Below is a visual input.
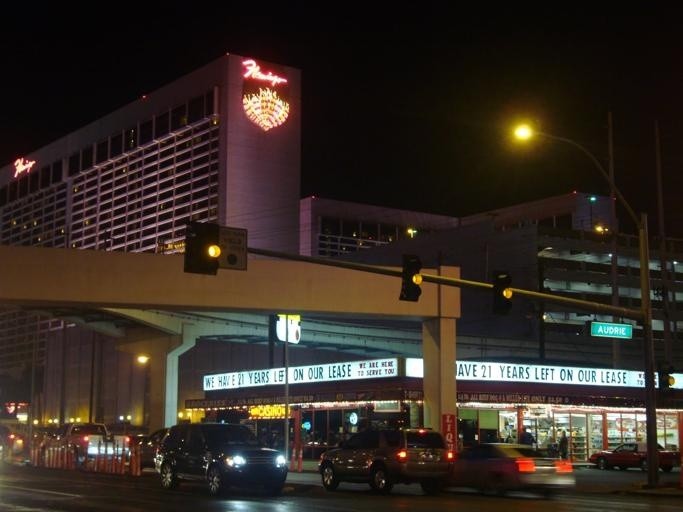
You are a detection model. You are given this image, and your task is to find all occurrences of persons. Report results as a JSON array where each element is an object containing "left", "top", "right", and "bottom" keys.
[
  {"left": 501, "top": 420, "right": 512, "bottom": 443},
  {"left": 520, "top": 426, "right": 537, "bottom": 445},
  {"left": 547, "top": 423, "right": 557, "bottom": 441},
  {"left": 558, "top": 431, "right": 569, "bottom": 459}
]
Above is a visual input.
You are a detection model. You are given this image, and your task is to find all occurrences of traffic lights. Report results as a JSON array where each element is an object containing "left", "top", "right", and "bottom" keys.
[
  {"left": 492, "top": 270, "right": 513, "bottom": 316},
  {"left": 399, "top": 254, "right": 423, "bottom": 302},
  {"left": 183, "top": 221, "right": 221, "bottom": 276},
  {"left": 658, "top": 361, "right": 675, "bottom": 393}
]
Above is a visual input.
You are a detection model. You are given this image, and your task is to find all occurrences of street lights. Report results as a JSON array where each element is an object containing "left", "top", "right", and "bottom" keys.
[
  {"left": 513, "top": 120, "right": 658, "bottom": 488},
  {"left": 594, "top": 220, "right": 638, "bottom": 240}
]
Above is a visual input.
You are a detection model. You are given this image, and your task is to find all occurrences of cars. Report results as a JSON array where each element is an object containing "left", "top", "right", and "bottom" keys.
[
  {"left": 0, "top": 419, "right": 287, "bottom": 496},
  {"left": 590, "top": 442, "right": 680, "bottom": 472},
  {"left": 318, "top": 426, "right": 576, "bottom": 496}
]
